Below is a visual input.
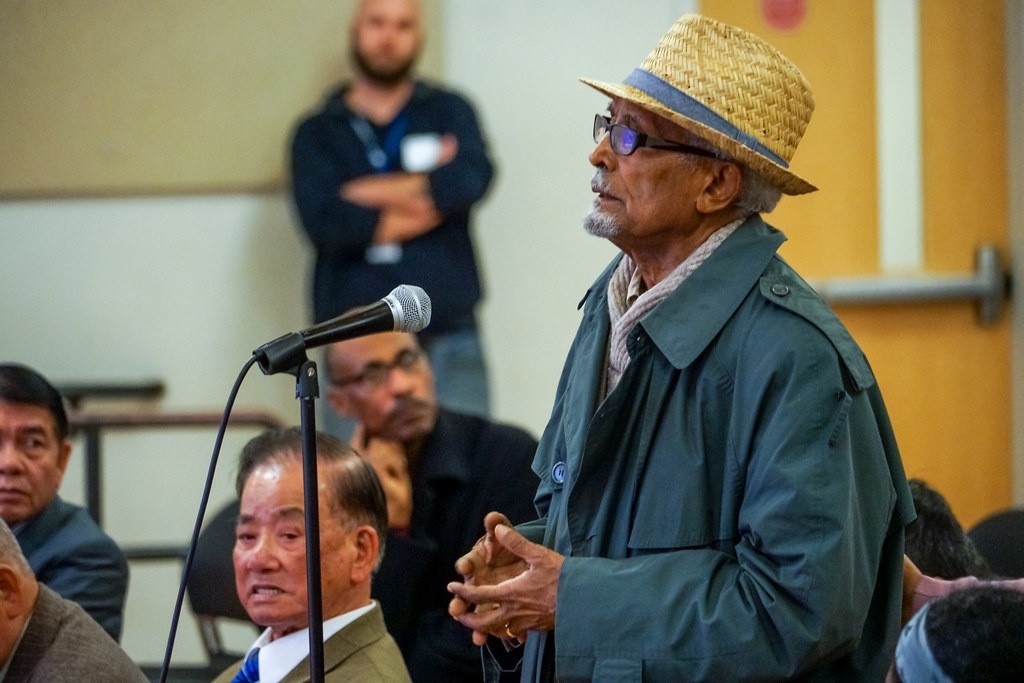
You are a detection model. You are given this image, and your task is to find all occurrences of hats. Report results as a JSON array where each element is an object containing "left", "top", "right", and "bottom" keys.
[{"left": 578, "top": 13, "right": 821, "bottom": 196}]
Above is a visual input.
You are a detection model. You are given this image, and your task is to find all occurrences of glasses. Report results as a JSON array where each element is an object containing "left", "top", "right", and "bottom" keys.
[
  {"left": 593, "top": 114, "right": 737, "bottom": 163},
  {"left": 337, "top": 352, "right": 420, "bottom": 384}
]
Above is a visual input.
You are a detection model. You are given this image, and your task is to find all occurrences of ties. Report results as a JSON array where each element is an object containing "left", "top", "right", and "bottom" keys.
[{"left": 230, "top": 647, "right": 259, "bottom": 683}]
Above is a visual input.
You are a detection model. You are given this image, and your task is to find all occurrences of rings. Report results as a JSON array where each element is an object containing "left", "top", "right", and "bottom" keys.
[{"left": 504, "top": 621, "right": 516, "bottom": 638}]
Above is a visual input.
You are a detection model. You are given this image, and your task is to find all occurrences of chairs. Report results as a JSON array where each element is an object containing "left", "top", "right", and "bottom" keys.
[{"left": 187, "top": 500, "right": 264, "bottom": 683}]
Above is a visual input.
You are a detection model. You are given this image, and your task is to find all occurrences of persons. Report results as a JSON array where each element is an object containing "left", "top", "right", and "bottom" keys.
[
  {"left": 209, "top": 425, "right": 411, "bottom": 683},
  {"left": 325, "top": 306, "right": 544, "bottom": 683},
  {"left": 286, "top": 0, "right": 497, "bottom": 446},
  {"left": 906, "top": 474, "right": 1024, "bottom": 683},
  {"left": 0, "top": 361, "right": 149, "bottom": 683},
  {"left": 446, "top": 13, "right": 918, "bottom": 683}
]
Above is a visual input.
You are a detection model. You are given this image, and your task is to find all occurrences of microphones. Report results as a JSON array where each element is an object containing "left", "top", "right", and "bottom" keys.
[{"left": 251, "top": 283, "right": 432, "bottom": 362}]
